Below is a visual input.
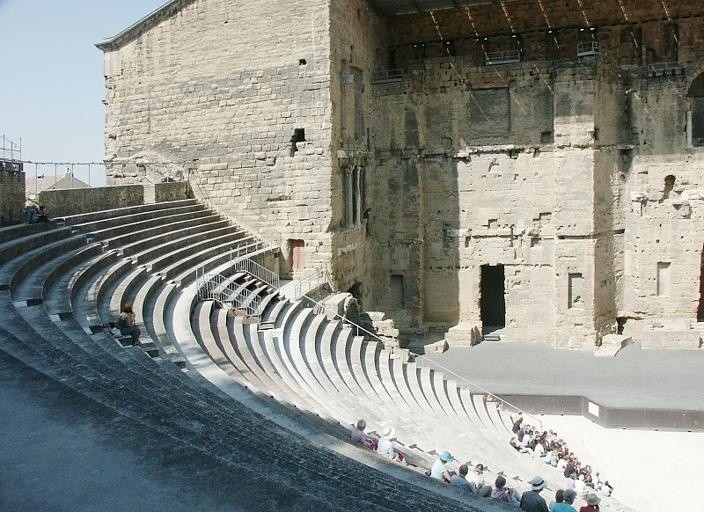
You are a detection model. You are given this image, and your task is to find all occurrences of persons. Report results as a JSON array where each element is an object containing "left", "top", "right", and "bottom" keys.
[
  {"left": 432, "top": 451, "right": 452, "bottom": 485},
  {"left": 113, "top": 304, "right": 141, "bottom": 346},
  {"left": 449, "top": 465, "right": 474, "bottom": 492},
  {"left": 491, "top": 477, "right": 521, "bottom": 508},
  {"left": 348, "top": 419, "right": 377, "bottom": 449},
  {"left": 510, "top": 416, "right": 613, "bottom": 512},
  {"left": 475, "top": 463, "right": 492, "bottom": 497},
  {"left": 376, "top": 426, "right": 405, "bottom": 463},
  {"left": 37, "top": 205, "right": 48, "bottom": 222}
]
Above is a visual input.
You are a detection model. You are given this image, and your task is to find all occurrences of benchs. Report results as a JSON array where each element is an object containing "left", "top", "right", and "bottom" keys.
[{"left": 0, "top": 197, "right": 621, "bottom": 512}]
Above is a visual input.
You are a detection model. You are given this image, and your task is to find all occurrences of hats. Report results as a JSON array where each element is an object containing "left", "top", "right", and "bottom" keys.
[
  {"left": 440, "top": 452, "right": 453, "bottom": 462},
  {"left": 383, "top": 427, "right": 394, "bottom": 439},
  {"left": 587, "top": 494, "right": 601, "bottom": 505},
  {"left": 532, "top": 476, "right": 544, "bottom": 491}
]
